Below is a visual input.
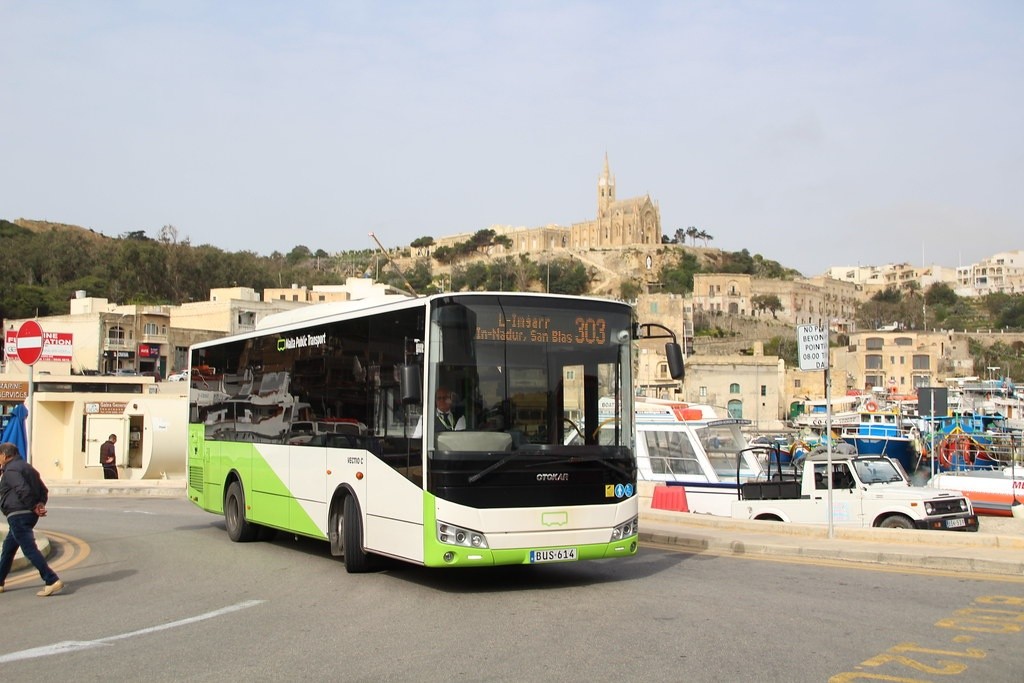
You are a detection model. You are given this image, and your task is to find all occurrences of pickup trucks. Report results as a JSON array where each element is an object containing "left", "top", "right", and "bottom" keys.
[{"left": 732, "top": 444, "right": 980, "bottom": 535}]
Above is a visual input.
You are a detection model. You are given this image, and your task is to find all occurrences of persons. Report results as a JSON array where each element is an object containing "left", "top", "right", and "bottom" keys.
[
  {"left": 100, "top": 434, "right": 118, "bottom": 480},
  {"left": 412, "top": 388, "right": 467, "bottom": 453},
  {"left": 0, "top": 443, "right": 64, "bottom": 596}
]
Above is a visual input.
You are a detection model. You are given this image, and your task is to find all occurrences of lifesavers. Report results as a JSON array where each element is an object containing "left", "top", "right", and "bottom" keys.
[
  {"left": 818, "top": 420, "right": 822, "bottom": 425},
  {"left": 958, "top": 437, "right": 971, "bottom": 462},
  {"left": 939, "top": 441, "right": 951, "bottom": 468},
  {"left": 866, "top": 401, "right": 878, "bottom": 413}
]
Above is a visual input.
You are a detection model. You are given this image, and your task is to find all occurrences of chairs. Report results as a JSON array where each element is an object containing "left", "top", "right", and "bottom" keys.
[
  {"left": 832, "top": 472, "right": 844, "bottom": 489},
  {"left": 816, "top": 472, "right": 827, "bottom": 489}
]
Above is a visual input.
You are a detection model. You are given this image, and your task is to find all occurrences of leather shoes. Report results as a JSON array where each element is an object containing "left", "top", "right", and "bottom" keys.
[
  {"left": 0, "top": 586, "right": 4, "bottom": 593},
  {"left": 37, "top": 580, "right": 64, "bottom": 596}
]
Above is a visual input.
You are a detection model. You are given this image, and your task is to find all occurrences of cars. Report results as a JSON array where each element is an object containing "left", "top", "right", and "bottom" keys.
[
  {"left": 111, "top": 368, "right": 138, "bottom": 376},
  {"left": 169, "top": 369, "right": 188, "bottom": 381},
  {"left": 137, "top": 371, "right": 161, "bottom": 383},
  {"left": 81, "top": 370, "right": 100, "bottom": 376}
]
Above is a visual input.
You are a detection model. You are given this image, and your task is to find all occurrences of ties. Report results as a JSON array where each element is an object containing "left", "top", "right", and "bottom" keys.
[{"left": 442, "top": 412, "right": 451, "bottom": 428}]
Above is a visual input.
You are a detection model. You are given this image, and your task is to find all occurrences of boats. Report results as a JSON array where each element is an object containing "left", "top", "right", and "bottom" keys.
[{"left": 563, "top": 376, "right": 1024, "bottom": 520}]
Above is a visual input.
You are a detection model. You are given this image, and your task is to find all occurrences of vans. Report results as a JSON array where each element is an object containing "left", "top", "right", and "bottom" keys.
[{"left": 289, "top": 417, "right": 367, "bottom": 447}]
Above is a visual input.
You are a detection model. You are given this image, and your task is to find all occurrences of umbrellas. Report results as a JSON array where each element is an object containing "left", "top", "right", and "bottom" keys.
[{"left": 0, "top": 404, "right": 28, "bottom": 463}]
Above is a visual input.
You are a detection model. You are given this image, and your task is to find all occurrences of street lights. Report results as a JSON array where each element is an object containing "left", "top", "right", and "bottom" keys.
[{"left": 115, "top": 312, "right": 130, "bottom": 372}]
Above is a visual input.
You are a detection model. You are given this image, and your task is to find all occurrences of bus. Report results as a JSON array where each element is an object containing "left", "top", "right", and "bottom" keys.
[{"left": 185, "top": 292, "right": 689, "bottom": 575}]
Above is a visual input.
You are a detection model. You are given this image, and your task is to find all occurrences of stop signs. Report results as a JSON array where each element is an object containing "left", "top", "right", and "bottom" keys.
[{"left": 15, "top": 321, "right": 44, "bottom": 366}]
